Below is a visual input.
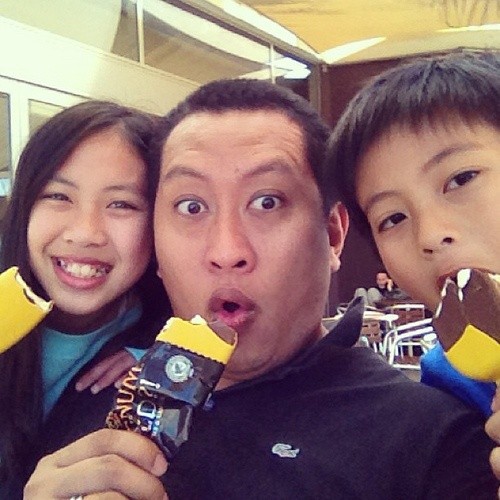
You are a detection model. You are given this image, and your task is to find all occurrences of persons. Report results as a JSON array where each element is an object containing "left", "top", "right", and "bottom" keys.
[
  {"left": 0, "top": 100, "right": 175, "bottom": 500},
  {"left": 326, "top": 45, "right": 499, "bottom": 499},
  {"left": 22, "top": 79, "right": 500, "bottom": 500},
  {"left": 353, "top": 270, "right": 398, "bottom": 312}
]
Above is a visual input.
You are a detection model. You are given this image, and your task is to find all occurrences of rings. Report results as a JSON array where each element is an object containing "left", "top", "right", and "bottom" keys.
[{"left": 68, "top": 493, "right": 87, "bottom": 500}]
